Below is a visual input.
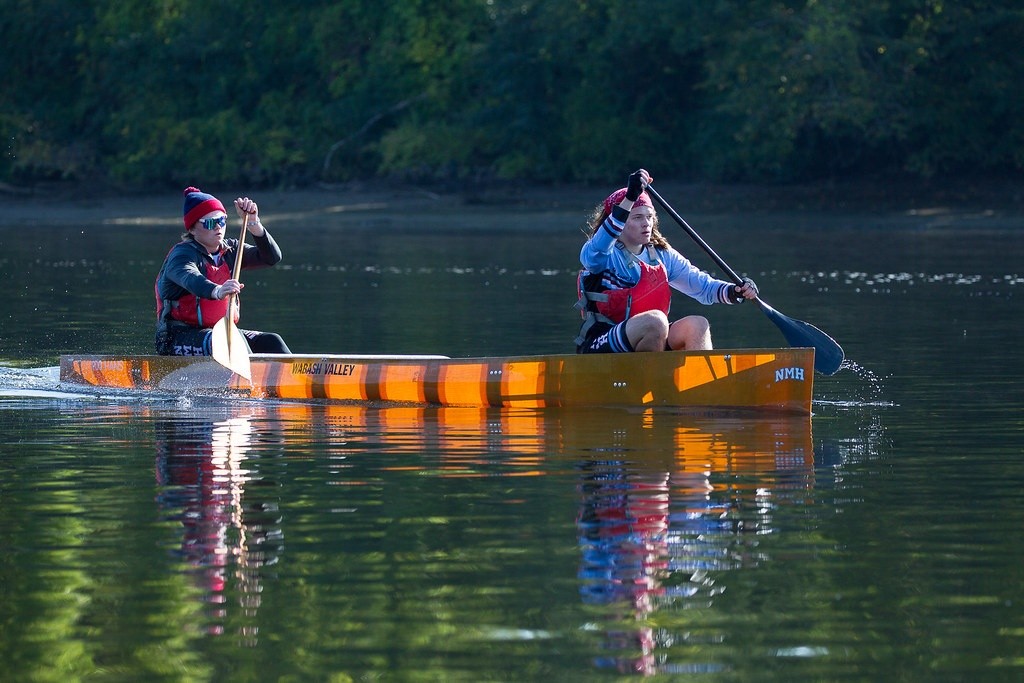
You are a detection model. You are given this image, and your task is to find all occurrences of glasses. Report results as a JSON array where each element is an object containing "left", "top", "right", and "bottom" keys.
[{"left": 199, "top": 215, "right": 227, "bottom": 230}]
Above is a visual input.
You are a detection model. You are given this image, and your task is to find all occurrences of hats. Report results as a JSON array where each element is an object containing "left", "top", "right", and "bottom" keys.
[{"left": 183, "top": 187, "right": 226, "bottom": 230}]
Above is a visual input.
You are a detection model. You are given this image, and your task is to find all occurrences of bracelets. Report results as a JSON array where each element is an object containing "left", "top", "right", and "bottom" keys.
[{"left": 247, "top": 220, "right": 259, "bottom": 226}]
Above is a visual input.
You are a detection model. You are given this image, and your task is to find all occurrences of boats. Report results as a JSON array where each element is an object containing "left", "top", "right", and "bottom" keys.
[{"left": 60, "top": 347, "right": 817, "bottom": 419}]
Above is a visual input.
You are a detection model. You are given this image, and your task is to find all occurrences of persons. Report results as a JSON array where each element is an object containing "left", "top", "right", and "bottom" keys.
[
  {"left": 155, "top": 187, "right": 292, "bottom": 354},
  {"left": 576, "top": 169, "right": 759, "bottom": 353}
]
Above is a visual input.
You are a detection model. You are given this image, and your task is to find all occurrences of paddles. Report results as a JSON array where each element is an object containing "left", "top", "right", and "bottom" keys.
[
  {"left": 209, "top": 205, "right": 256, "bottom": 383},
  {"left": 645, "top": 182, "right": 846, "bottom": 378}
]
